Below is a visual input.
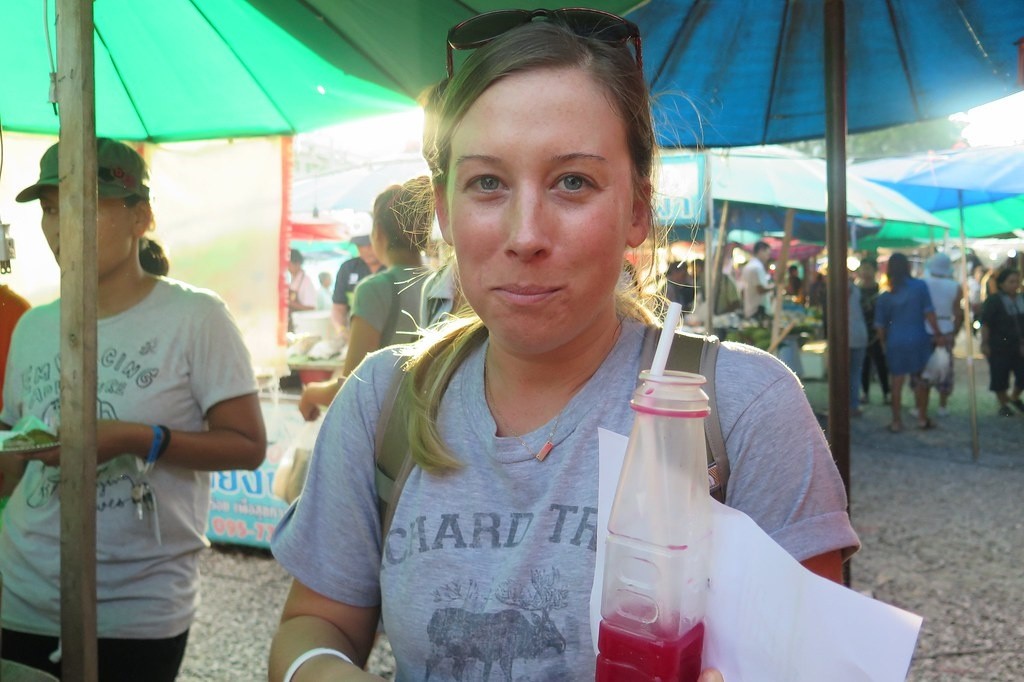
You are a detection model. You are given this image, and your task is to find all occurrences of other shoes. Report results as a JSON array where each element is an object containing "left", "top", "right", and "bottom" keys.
[
  {"left": 891, "top": 425, "right": 900, "bottom": 432},
  {"left": 884, "top": 392, "right": 892, "bottom": 403},
  {"left": 1009, "top": 397, "right": 1024, "bottom": 411},
  {"left": 859, "top": 393, "right": 868, "bottom": 403},
  {"left": 937, "top": 406, "right": 950, "bottom": 416},
  {"left": 1000, "top": 407, "right": 1012, "bottom": 416},
  {"left": 919, "top": 420, "right": 928, "bottom": 427},
  {"left": 909, "top": 408, "right": 920, "bottom": 418}
]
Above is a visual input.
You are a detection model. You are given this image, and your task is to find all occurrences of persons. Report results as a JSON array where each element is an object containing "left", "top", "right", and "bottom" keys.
[
  {"left": 285, "top": 175, "right": 1024, "bottom": 435},
  {"left": 0, "top": 136, "right": 267, "bottom": 682},
  {"left": 265, "top": 22, "right": 861, "bottom": 682}
]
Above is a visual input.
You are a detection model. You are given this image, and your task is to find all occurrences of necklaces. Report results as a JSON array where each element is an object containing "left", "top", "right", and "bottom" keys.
[{"left": 486, "top": 353, "right": 564, "bottom": 462}]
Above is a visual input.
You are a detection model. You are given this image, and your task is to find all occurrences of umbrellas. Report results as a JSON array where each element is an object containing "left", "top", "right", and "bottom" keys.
[
  {"left": 620, "top": 0, "right": 1024, "bottom": 251},
  {"left": 0, "top": 0, "right": 648, "bottom": 143}
]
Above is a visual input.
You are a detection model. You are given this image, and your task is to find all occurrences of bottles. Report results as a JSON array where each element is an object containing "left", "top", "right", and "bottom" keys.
[{"left": 595, "top": 370, "right": 711, "bottom": 682}]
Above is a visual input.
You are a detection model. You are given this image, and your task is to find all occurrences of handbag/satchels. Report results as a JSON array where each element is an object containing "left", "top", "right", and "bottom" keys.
[
  {"left": 847, "top": 286, "right": 869, "bottom": 348},
  {"left": 921, "top": 345, "right": 950, "bottom": 384},
  {"left": 272, "top": 443, "right": 314, "bottom": 505},
  {"left": 699, "top": 271, "right": 739, "bottom": 315}
]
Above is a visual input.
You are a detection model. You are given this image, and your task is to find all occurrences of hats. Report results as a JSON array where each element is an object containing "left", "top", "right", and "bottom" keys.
[
  {"left": 348, "top": 210, "right": 374, "bottom": 245},
  {"left": 928, "top": 253, "right": 954, "bottom": 274},
  {"left": 15, "top": 138, "right": 149, "bottom": 199}
]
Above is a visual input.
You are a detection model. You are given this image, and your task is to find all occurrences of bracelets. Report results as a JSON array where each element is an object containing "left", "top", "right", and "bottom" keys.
[
  {"left": 157, "top": 424, "right": 171, "bottom": 456},
  {"left": 136, "top": 423, "right": 162, "bottom": 475},
  {"left": 277, "top": 648, "right": 355, "bottom": 682}
]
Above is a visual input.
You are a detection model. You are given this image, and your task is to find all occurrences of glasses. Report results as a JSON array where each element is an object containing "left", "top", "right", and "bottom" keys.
[{"left": 447, "top": 7, "right": 644, "bottom": 79}]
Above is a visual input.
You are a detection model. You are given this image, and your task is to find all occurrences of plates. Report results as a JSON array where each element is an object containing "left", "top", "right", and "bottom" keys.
[{"left": 0, "top": 430, "right": 60, "bottom": 453}]
